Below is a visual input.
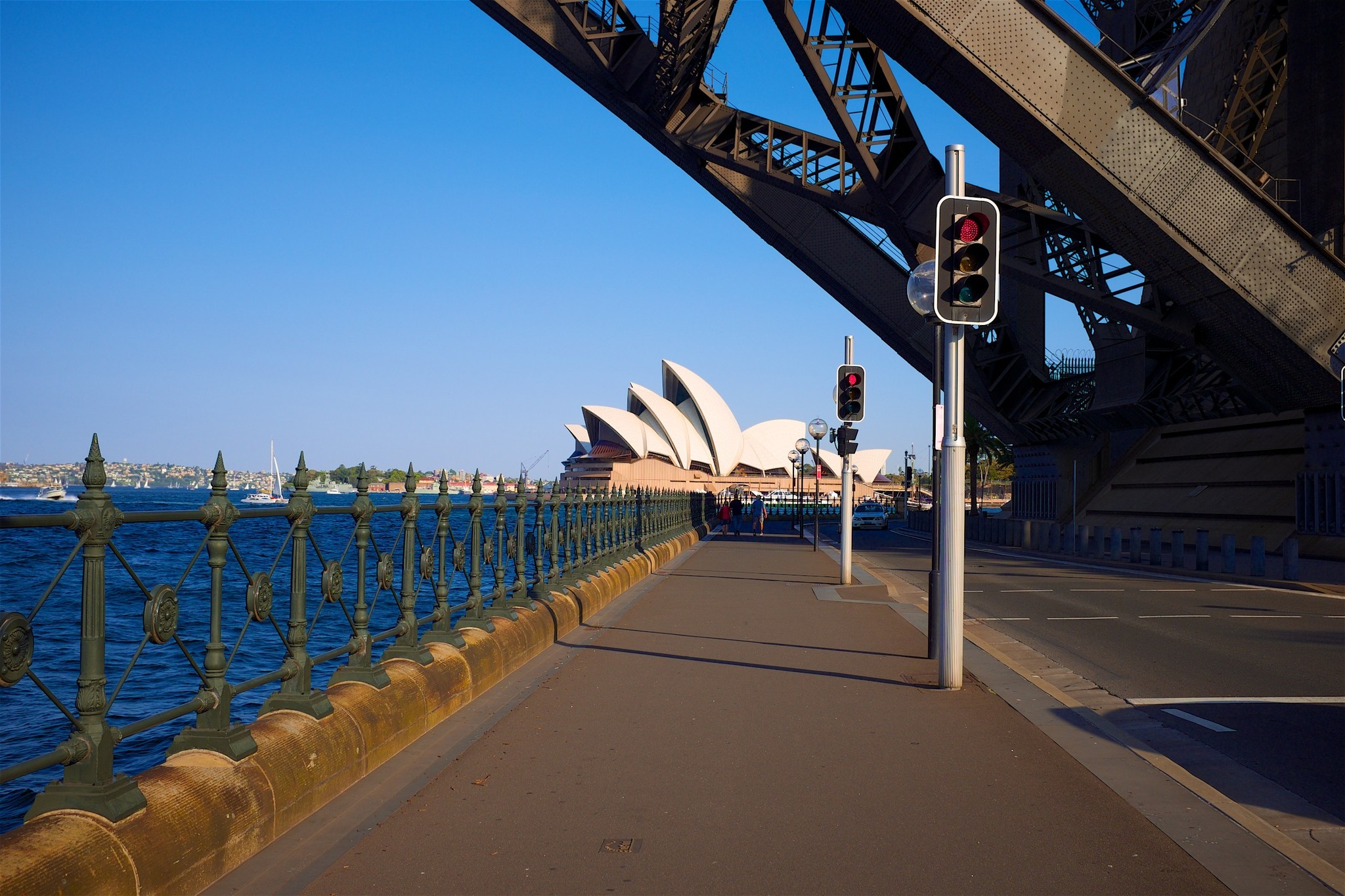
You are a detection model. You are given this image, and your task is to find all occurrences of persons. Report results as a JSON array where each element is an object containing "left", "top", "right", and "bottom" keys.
[
  {"left": 729, "top": 494, "right": 744, "bottom": 536},
  {"left": 751, "top": 495, "right": 768, "bottom": 537},
  {"left": 717, "top": 501, "right": 733, "bottom": 537}
]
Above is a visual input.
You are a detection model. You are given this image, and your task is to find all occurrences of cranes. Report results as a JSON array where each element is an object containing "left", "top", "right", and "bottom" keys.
[{"left": 520, "top": 450, "right": 549, "bottom": 484}]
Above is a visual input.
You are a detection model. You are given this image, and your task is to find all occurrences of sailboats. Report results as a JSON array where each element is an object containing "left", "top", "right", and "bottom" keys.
[{"left": 241, "top": 440, "right": 290, "bottom": 503}]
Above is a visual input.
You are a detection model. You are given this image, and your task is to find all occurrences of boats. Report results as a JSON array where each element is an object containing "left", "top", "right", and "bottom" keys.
[
  {"left": 327, "top": 489, "right": 340, "bottom": 494},
  {"left": 36, "top": 485, "right": 67, "bottom": 500}
]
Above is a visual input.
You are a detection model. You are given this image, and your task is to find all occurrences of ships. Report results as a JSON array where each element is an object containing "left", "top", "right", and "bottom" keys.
[{"left": 288, "top": 471, "right": 358, "bottom": 492}]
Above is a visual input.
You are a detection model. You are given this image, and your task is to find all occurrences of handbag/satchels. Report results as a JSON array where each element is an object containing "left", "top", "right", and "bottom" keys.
[{"left": 717, "top": 507, "right": 724, "bottom": 519}]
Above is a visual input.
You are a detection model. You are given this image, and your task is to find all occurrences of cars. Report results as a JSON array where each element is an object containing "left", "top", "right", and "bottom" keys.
[{"left": 852, "top": 503, "right": 889, "bottom": 530}]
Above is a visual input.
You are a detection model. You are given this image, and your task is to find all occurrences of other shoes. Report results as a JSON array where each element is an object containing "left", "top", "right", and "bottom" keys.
[
  {"left": 738, "top": 533, "right": 740, "bottom": 536},
  {"left": 760, "top": 532, "right": 763, "bottom": 537},
  {"left": 723, "top": 532, "right": 724, "bottom": 536},
  {"left": 735, "top": 535, "right": 737, "bottom": 537},
  {"left": 753, "top": 533, "right": 757, "bottom": 536},
  {"left": 725, "top": 532, "right": 727, "bottom": 536}
]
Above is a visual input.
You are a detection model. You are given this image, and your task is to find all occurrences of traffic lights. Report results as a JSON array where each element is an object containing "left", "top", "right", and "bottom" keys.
[
  {"left": 933, "top": 193, "right": 1000, "bottom": 326},
  {"left": 907, "top": 466, "right": 912, "bottom": 491},
  {"left": 833, "top": 364, "right": 865, "bottom": 457}
]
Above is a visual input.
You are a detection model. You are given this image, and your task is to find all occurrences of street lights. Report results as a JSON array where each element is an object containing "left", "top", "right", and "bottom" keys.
[
  {"left": 908, "top": 260, "right": 944, "bottom": 659},
  {"left": 788, "top": 417, "right": 827, "bottom": 551}
]
